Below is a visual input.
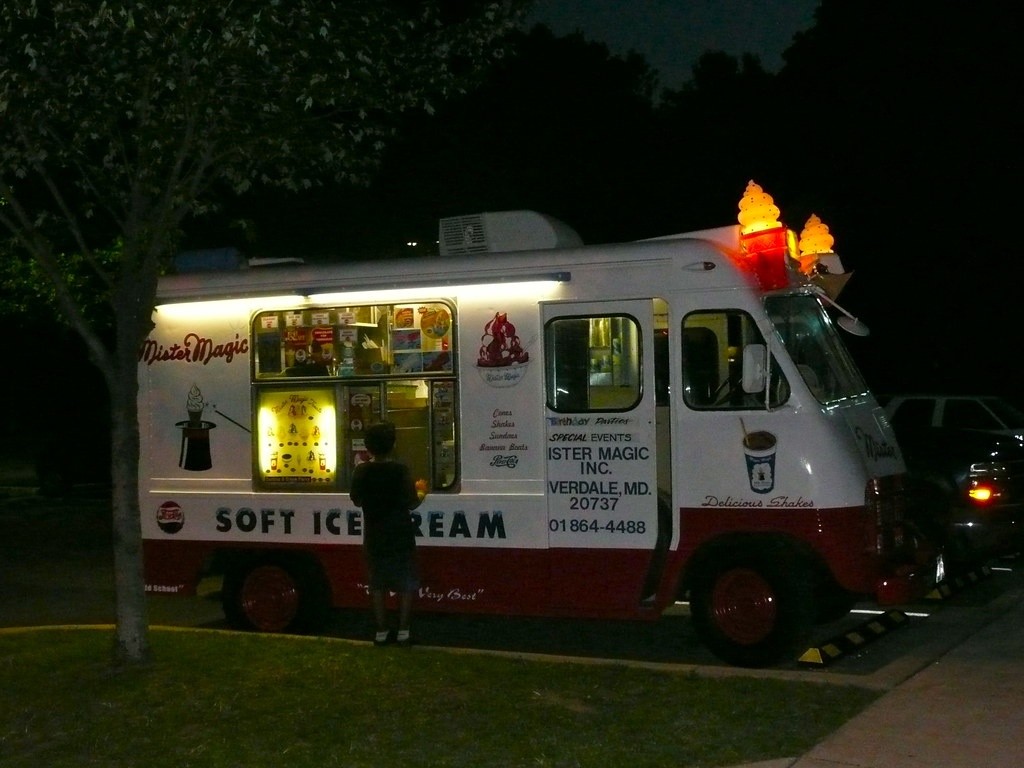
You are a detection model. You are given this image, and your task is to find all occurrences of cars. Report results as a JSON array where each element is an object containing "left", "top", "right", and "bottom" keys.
[{"left": 888, "top": 393, "right": 1023, "bottom": 599}]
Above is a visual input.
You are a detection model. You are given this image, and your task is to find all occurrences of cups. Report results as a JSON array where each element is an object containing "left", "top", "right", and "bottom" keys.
[{"left": 742, "top": 430, "right": 777, "bottom": 494}]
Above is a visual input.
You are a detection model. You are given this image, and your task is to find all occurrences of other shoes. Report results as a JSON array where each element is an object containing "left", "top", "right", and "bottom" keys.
[{"left": 374, "top": 629, "right": 423, "bottom": 646}]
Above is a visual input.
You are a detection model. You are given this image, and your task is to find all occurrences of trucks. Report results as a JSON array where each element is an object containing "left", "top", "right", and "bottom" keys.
[{"left": 134, "top": 181, "right": 957, "bottom": 670}]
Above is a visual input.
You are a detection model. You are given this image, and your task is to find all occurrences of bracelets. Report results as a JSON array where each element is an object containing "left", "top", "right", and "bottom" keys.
[{"left": 417, "top": 492, "right": 425, "bottom": 502}]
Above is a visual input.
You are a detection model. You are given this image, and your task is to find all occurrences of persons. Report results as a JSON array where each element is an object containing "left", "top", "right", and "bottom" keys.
[{"left": 350, "top": 421, "right": 427, "bottom": 647}]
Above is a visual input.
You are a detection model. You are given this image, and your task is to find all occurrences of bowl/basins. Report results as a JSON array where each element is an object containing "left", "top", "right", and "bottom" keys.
[{"left": 473, "top": 358, "right": 532, "bottom": 388}]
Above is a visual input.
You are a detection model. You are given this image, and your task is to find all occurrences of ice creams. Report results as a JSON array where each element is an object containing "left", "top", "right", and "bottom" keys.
[
  {"left": 799, "top": 213, "right": 834, "bottom": 273},
  {"left": 737, "top": 179, "right": 788, "bottom": 291}
]
